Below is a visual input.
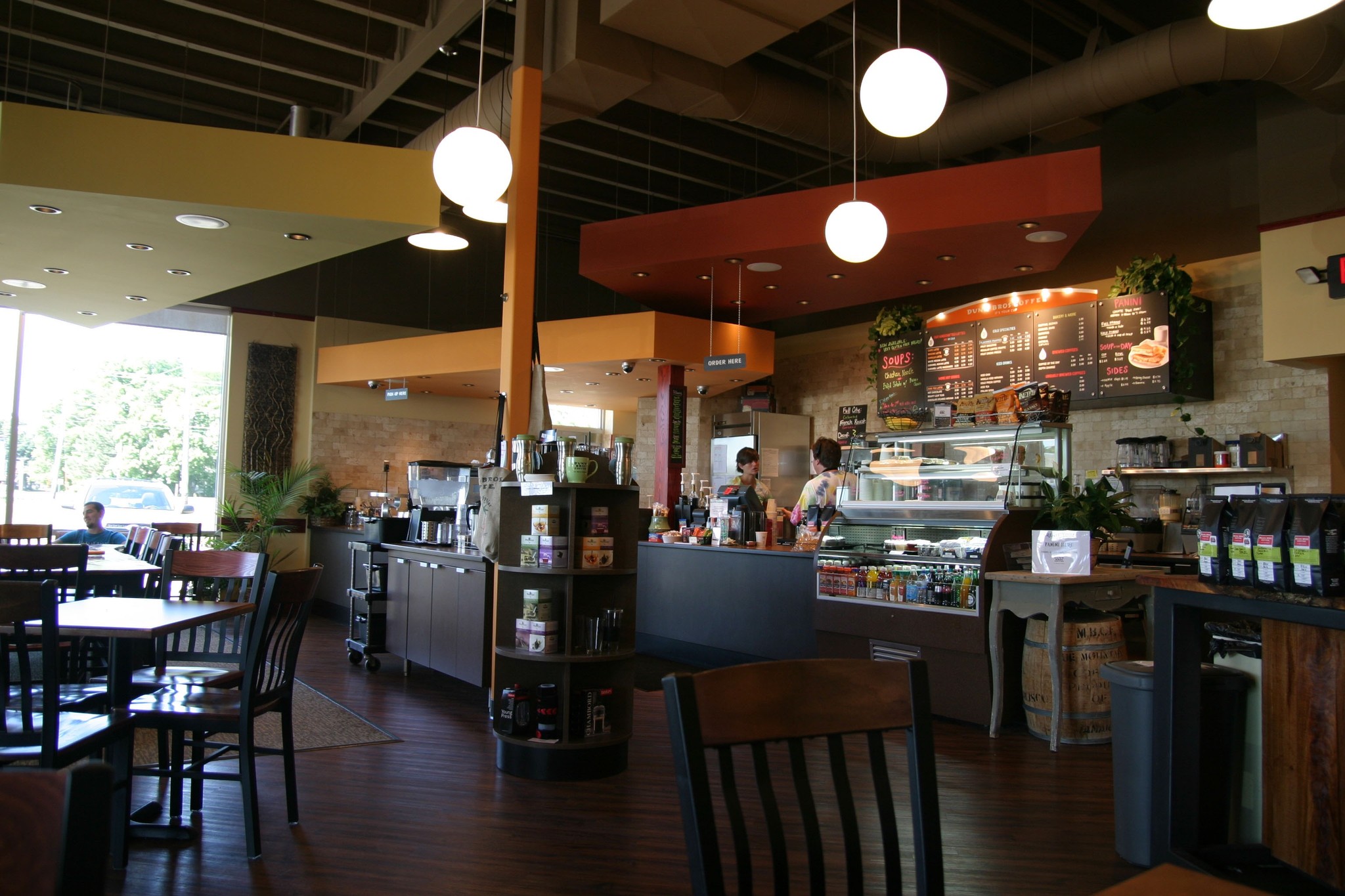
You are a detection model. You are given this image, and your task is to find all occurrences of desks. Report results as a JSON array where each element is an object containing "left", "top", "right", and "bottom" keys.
[
  {"left": 986, "top": 565, "right": 1164, "bottom": 753},
  {"left": 0, "top": 596, "right": 258, "bottom": 873},
  {"left": 0, "top": 544, "right": 164, "bottom": 714},
  {"left": 1141, "top": 573, "right": 1345, "bottom": 896}
]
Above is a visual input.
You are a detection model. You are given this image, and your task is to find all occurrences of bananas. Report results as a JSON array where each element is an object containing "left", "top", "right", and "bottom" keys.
[{"left": 884, "top": 417, "right": 917, "bottom": 430}]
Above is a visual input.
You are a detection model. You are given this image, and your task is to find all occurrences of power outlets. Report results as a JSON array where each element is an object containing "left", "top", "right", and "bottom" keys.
[{"left": 383, "top": 460, "right": 389, "bottom": 472}]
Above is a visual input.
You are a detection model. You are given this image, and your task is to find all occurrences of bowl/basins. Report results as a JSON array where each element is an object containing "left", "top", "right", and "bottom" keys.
[
  {"left": 662, "top": 535, "right": 682, "bottom": 543},
  {"left": 746, "top": 541, "right": 756, "bottom": 546}
]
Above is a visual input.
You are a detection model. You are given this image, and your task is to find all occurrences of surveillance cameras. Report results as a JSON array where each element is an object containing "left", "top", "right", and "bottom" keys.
[
  {"left": 696, "top": 386, "right": 709, "bottom": 395},
  {"left": 621, "top": 361, "right": 635, "bottom": 374},
  {"left": 367, "top": 380, "right": 380, "bottom": 389}
]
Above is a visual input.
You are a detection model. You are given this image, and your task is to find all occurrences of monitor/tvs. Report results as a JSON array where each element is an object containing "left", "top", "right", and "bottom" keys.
[{"left": 717, "top": 484, "right": 768, "bottom": 532}]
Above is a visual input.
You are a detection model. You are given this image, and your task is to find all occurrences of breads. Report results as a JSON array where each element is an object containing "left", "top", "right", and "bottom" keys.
[{"left": 1130, "top": 343, "right": 1165, "bottom": 365}]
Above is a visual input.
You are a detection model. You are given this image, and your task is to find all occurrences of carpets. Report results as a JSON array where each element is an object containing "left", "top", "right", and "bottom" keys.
[{"left": 0, "top": 623, "right": 404, "bottom": 766}]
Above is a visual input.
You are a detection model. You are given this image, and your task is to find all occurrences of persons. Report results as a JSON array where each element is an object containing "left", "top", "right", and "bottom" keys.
[
  {"left": 781, "top": 437, "right": 857, "bottom": 525},
  {"left": 52, "top": 501, "right": 127, "bottom": 545},
  {"left": 726, "top": 447, "right": 774, "bottom": 506}
]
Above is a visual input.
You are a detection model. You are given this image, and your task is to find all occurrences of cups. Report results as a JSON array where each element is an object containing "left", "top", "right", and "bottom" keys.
[
  {"left": 512, "top": 435, "right": 542, "bottom": 482},
  {"left": 614, "top": 437, "right": 635, "bottom": 486},
  {"left": 437, "top": 504, "right": 469, "bottom": 547},
  {"left": 536, "top": 683, "right": 561, "bottom": 739},
  {"left": 574, "top": 607, "right": 624, "bottom": 655},
  {"left": 575, "top": 445, "right": 609, "bottom": 458},
  {"left": 755, "top": 531, "right": 768, "bottom": 547},
  {"left": 766, "top": 498, "right": 777, "bottom": 546},
  {"left": 987, "top": 446, "right": 1005, "bottom": 464},
  {"left": 855, "top": 461, "right": 893, "bottom": 500},
  {"left": 566, "top": 456, "right": 598, "bottom": 483},
  {"left": 557, "top": 438, "right": 578, "bottom": 483},
  {"left": 998, "top": 481, "right": 1043, "bottom": 507},
  {"left": 1154, "top": 325, "right": 1168, "bottom": 342}
]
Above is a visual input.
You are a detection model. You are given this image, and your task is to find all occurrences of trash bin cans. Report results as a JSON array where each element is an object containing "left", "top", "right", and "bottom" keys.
[{"left": 1098, "top": 654, "right": 1255, "bottom": 871}]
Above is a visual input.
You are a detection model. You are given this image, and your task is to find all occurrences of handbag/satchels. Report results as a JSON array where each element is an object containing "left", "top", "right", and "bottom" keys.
[{"left": 472, "top": 467, "right": 510, "bottom": 563}]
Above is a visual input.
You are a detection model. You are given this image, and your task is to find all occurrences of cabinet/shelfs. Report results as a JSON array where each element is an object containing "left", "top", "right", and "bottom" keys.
[
  {"left": 837, "top": 422, "right": 1073, "bottom": 523},
  {"left": 492, "top": 450, "right": 641, "bottom": 782},
  {"left": 381, "top": 541, "right": 494, "bottom": 688}
]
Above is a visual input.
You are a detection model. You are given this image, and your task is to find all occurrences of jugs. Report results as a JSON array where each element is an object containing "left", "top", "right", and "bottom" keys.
[
  {"left": 345, "top": 505, "right": 365, "bottom": 526},
  {"left": 466, "top": 501, "right": 482, "bottom": 549},
  {"left": 1115, "top": 436, "right": 1172, "bottom": 469}
]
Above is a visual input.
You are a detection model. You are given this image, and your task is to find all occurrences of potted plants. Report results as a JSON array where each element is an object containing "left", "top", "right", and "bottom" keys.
[
  {"left": 864, "top": 304, "right": 924, "bottom": 403},
  {"left": 1033, "top": 463, "right": 1143, "bottom": 573},
  {"left": 205, "top": 458, "right": 353, "bottom": 617},
  {"left": 1109, "top": 252, "right": 1210, "bottom": 441}
]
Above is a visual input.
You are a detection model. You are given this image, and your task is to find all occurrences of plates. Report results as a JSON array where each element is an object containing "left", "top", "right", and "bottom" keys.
[{"left": 1128, "top": 346, "right": 1169, "bottom": 368}]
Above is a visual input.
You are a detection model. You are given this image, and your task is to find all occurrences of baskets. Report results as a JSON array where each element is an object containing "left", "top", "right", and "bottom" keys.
[{"left": 878, "top": 408, "right": 930, "bottom": 431}]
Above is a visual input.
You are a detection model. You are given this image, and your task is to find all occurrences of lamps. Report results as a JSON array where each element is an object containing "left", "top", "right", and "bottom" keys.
[
  {"left": 408, "top": 206, "right": 469, "bottom": 251},
  {"left": 860, "top": 0, "right": 948, "bottom": 139},
  {"left": 824, "top": 0, "right": 888, "bottom": 263},
  {"left": 463, "top": 193, "right": 508, "bottom": 223},
  {"left": 433, "top": 0, "right": 512, "bottom": 208},
  {"left": 1295, "top": 254, "right": 1345, "bottom": 300}
]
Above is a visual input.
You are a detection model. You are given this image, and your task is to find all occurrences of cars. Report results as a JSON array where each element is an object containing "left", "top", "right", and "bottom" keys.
[{"left": 61, "top": 478, "right": 194, "bottom": 530}]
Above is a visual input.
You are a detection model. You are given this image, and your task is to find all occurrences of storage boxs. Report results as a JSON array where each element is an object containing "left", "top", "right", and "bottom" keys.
[
  {"left": 514, "top": 504, "right": 617, "bottom": 740},
  {"left": 355, "top": 517, "right": 410, "bottom": 645}
]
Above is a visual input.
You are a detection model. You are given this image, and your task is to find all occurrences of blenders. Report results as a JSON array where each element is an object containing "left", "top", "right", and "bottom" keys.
[{"left": 1156, "top": 488, "right": 1187, "bottom": 555}]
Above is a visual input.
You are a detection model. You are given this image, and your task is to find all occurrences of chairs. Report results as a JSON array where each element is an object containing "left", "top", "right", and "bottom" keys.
[
  {"left": 663, "top": 660, "right": 946, "bottom": 896},
  {"left": 0, "top": 523, "right": 324, "bottom": 896}
]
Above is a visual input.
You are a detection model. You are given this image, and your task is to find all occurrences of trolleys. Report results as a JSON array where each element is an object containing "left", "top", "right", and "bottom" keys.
[{"left": 344, "top": 541, "right": 392, "bottom": 672}]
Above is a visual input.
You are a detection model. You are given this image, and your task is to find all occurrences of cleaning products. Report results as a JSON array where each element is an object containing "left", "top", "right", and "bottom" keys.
[
  {"left": 688, "top": 473, "right": 700, "bottom": 505},
  {"left": 706, "top": 486, "right": 715, "bottom": 510},
  {"left": 698, "top": 479, "right": 708, "bottom": 507},
  {"left": 646, "top": 495, "right": 654, "bottom": 509},
  {"left": 680, "top": 473, "right": 690, "bottom": 504}
]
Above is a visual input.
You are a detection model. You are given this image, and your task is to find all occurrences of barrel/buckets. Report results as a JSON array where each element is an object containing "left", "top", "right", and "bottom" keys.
[{"left": 1023, "top": 612, "right": 1127, "bottom": 747}]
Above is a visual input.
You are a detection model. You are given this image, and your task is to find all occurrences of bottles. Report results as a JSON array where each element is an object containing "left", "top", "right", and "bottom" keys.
[
  {"left": 818, "top": 559, "right": 980, "bottom": 610},
  {"left": 648, "top": 516, "right": 670, "bottom": 534}
]
